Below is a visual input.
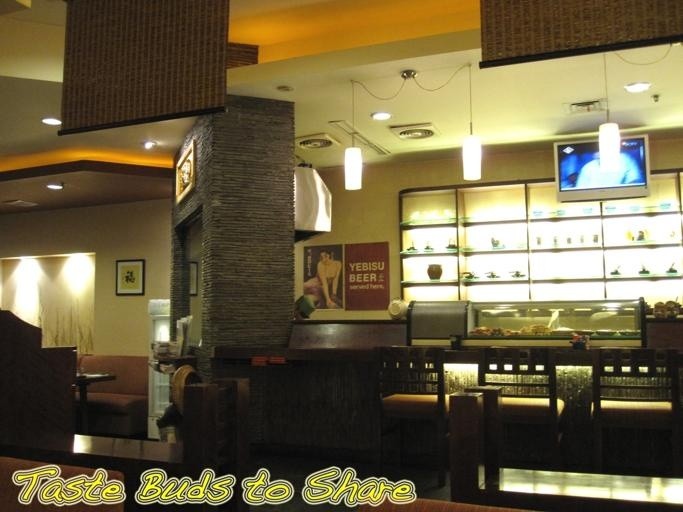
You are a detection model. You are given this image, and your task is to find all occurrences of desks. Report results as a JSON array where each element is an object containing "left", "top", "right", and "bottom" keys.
[
  {"left": 3, "top": 427, "right": 187, "bottom": 467},
  {"left": 71, "top": 372, "right": 116, "bottom": 431}
]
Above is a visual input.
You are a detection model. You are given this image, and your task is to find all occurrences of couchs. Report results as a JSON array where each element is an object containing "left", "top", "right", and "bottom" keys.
[{"left": 73, "top": 355, "right": 149, "bottom": 438}]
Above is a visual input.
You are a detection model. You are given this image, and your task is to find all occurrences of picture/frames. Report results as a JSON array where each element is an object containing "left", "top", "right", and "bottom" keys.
[
  {"left": 174, "top": 140, "right": 195, "bottom": 205},
  {"left": 115, "top": 260, "right": 144, "bottom": 296},
  {"left": 188, "top": 261, "right": 199, "bottom": 295}
]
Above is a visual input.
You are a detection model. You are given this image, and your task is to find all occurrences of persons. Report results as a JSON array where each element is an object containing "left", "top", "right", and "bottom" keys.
[
  {"left": 304, "top": 247, "right": 342, "bottom": 309},
  {"left": 574, "top": 143, "right": 645, "bottom": 189}
]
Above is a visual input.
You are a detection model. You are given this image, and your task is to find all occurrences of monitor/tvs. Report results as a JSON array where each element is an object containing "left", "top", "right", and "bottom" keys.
[{"left": 553, "top": 134, "right": 650, "bottom": 202}]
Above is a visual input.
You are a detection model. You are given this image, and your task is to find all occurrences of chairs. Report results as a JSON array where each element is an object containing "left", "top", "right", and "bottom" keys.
[{"left": 375, "top": 345, "right": 682, "bottom": 495}]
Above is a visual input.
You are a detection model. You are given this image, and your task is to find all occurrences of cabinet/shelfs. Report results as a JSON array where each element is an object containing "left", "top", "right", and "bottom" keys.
[{"left": 397, "top": 167, "right": 683, "bottom": 306}]
